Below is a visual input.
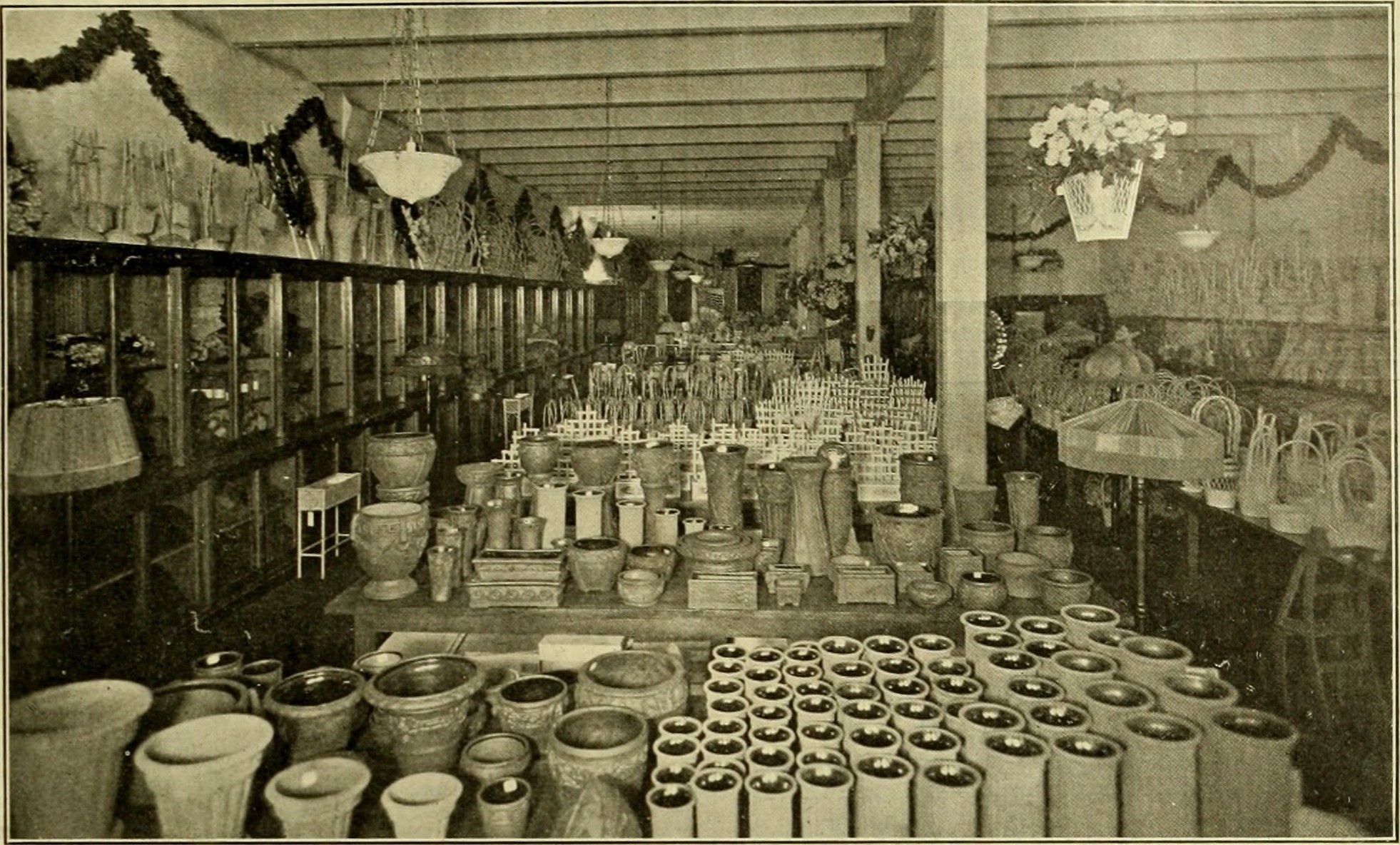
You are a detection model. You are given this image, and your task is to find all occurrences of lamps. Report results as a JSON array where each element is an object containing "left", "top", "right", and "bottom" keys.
[
  {"left": 1018, "top": 171, "right": 1045, "bottom": 270},
  {"left": 669, "top": 191, "right": 692, "bottom": 282},
  {"left": 686, "top": 202, "right": 705, "bottom": 283},
  {"left": 701, "top": 237, "right": 715, "bottom": 286},
  {"left": 1172, "top": 63, "right": 1223, "bottom": 253},
  {"left": 8, "top": 396, "right": 145, "bottom": 691},
  {"left": 590, "top": 77, "right": 630, "bottom": 259},
  {"left": 648, "top": 160, "right": 676, "bottom": 272},
  {"left": 357, "top": 10, "right": 464, "bottom": 205},
  {"left": 1055, "top": 394, "right": 1226, "bottom": 635}
]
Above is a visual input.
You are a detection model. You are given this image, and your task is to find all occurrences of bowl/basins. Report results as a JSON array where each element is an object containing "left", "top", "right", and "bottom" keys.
[
  {"left": 517, "top": 435, "right": 560, "bottom": 483},
  {"left": 368, "top": 432, "right": 437, "bottom": 502},
  {"left": 574, "top": 650, "right": 689, "bottom": 742},
  {"left": 940, "top": 520, "right": 1075, "bottom": 610},
  {"left": 1035, "top": 569, "right": 1094, "bottom": 612},
  {"left": 262, "top": 653, "right": 485, "bottom": 839},
  {"left": 907, "top": 578, "right": 951, "bottom": 608},
  {"left": 873, "top": 502, "right": 942, "bottom": 566},
  {"left": 460, "top": 732, "right": 531, "bottom": 787},
  {"left": 570, "top": 440, "right": 623, "bottom": 487},
  {"left": 9, "top": 677, "right": 249, "bottom": 841},
  {"left": 569, "top": 537, "right": 676, "bottom": 607},
  {"left": 498, "top": 675, "right": 567, "bottom": 756},
  {"left": 547, "top": 705, "right": 648, "bottom": 805}
]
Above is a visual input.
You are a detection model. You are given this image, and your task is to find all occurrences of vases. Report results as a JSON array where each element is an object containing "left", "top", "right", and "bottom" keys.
[
  {"left": 899, "top": 253, "right": 930, "bottom": 278},
  {"left": 1055, "top": 155, "right": 1145, "bottom": 242}
]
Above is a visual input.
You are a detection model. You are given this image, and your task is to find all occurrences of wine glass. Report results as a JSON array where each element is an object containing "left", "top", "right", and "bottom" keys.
[{"left": 352, "top": 502, "right": 429, "bottom": 600}]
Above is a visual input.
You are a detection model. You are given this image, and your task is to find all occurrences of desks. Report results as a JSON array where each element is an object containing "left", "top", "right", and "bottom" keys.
[{"left": 113, "top": 348, "right": 1393, "bottom": 837}]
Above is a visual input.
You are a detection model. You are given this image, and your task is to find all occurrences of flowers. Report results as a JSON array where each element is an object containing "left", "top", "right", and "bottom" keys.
[
  {"left": 774, "top": 201, "right": 938, "bottom": 319},
  {"left": 1023, "top": 75, "right": 1191, "bottom": 189}
]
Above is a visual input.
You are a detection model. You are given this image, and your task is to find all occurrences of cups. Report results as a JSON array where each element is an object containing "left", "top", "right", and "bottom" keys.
[
  {"left": 192, "top": 651, "right": 283, "bottom": 696},
  {"left": 536, "top": 482, "right": 706, "bottom": 550},
  {"left": 899, "top": 453, "right": 1041, "bottom": 551},
  {"left": 428, "top": 504, "right": 479, "bottom": 602},
  {"left": 477, "top": 777, "right": 531, "bottom": 839},
  {"left": 819, "top": 635, "right": 982, "bottom": 839},
  {"left": 742, "top": 641, "right": 854, "bottom": 839},
  {"left": 1061, "top": 605, "right": 1301, "bottom": 837},
  {"left": 910, "top": 610, "right": 1124, "bottom": 839},
  {"left": 648, "top": 643, "right": 749, "bottom": 839}
]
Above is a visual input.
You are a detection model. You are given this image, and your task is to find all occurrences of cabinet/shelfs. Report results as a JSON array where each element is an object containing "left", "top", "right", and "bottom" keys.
[{"left": 3, "top": 229, "right": 627, "bottom": 709}]
